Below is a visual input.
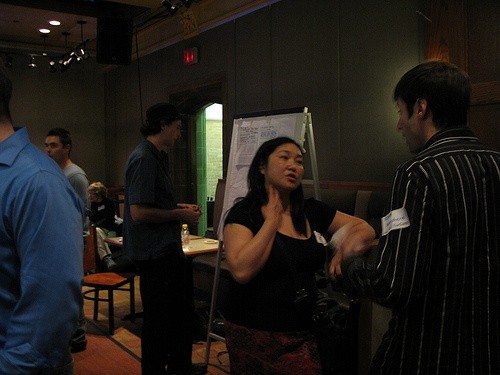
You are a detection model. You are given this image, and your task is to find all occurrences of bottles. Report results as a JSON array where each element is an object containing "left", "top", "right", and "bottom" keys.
[{"left": 181, "top": 224, "right": 189, "bottom": 252}]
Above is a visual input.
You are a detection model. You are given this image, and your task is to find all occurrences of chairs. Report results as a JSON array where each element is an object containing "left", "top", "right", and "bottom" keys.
[{"left": 82, "top": 254, "right": 136, "bottom": 334}]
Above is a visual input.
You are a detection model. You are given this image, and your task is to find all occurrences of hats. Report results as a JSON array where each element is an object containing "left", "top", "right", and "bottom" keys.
[{"left": 146, "top": 102, "right": 182, "bottom": 122}]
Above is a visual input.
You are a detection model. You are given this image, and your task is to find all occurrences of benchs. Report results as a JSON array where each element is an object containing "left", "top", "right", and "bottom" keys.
[{"left": 191, "top": 177, "right": 408, "bottom": 314}]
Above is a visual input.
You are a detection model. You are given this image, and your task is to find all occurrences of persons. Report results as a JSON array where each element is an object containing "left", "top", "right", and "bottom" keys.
[
  {"left": 45, "top": 128, "right": 91, "bottom": 354},
  {"left": 222, "top": 137, "right": 375, "bottom": 375},
  {"left": 122, "top": 103, "right": 207, "bottom": 375},
  {"left": 0, "top": 70, "right": 85, "bottom": 375},
  {"left": 88, "top": 182, "right": 118, "bottom": 271},
  {"left": 348, "top": 61, "right": 500, "bottom": 375}
]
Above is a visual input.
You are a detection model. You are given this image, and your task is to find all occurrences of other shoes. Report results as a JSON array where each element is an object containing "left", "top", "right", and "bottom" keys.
[
  {"left": 189, "top": 363, "right": 207, "bottom": 375},
  {"left": 103, "top": 256, "right": 117, "bottom": 270},
  {"left": 71, "top": 335, "right": 85, "bottom": 351}
]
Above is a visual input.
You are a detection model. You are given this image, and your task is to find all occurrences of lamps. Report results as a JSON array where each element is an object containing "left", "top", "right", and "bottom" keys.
[
  {"left": 29, "top": 20, "right": 91, "bottom": 73},
  {"left": 162, "top": 0, "right": 178, "bottom": 13},
  {"left": 180, "top": 0, "right": 193, "bottom": 8}
]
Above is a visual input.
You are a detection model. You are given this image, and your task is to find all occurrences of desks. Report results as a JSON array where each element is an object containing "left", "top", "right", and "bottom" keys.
[{"left": 105, "top": 234, "right": 226, "bottom": 254}]
